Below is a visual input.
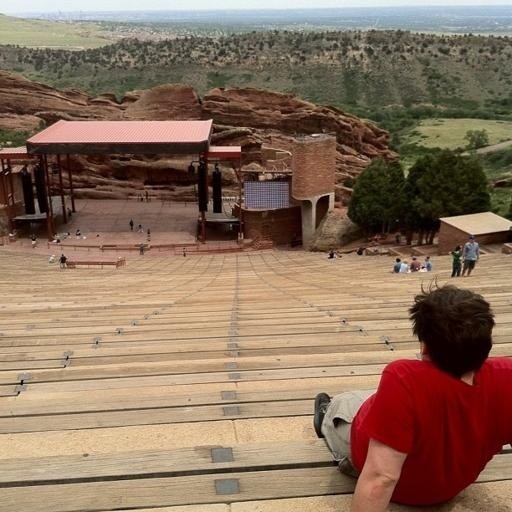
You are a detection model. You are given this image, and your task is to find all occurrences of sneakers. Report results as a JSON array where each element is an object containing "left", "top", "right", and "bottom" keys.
[{"left": 314, "top": 393, "right": 331, "bottom": 438}]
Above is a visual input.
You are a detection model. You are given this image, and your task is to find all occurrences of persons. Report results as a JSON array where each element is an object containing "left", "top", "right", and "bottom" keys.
[
  {"left": 129, "top": 217, "right": 143, "bottom": 233},
  {"left": 314, "top": 285, "right": 512, "bottom": 512},
  {"left": 75, "top": 229, "right": 81, "bottom": 239},
  {"left": 390, "top": 235, "right": 480, "bottom": 277},
  {"left": 326, "top": 245, "right": 366, "bottom": 259},
  {"left": 29, "top": 233, "right": 40, "bottom": 249},
  {"left": 139, "top": 242, "right": 144, "bottom": 254},
  {"left": 182, "top": 248, "right": 187, "bottom": 258},
  {"left": 58, "top": 254, "right": 68, "bottom": 269},
  {"left": 47, "top": 232, "right": 57, "bottom": 242},
  {"left": 140, "top": 190, "right": 148, "bottom": 202},
  {"left": 67, "top": 207, "right": 72, "bottom": 221}
]
